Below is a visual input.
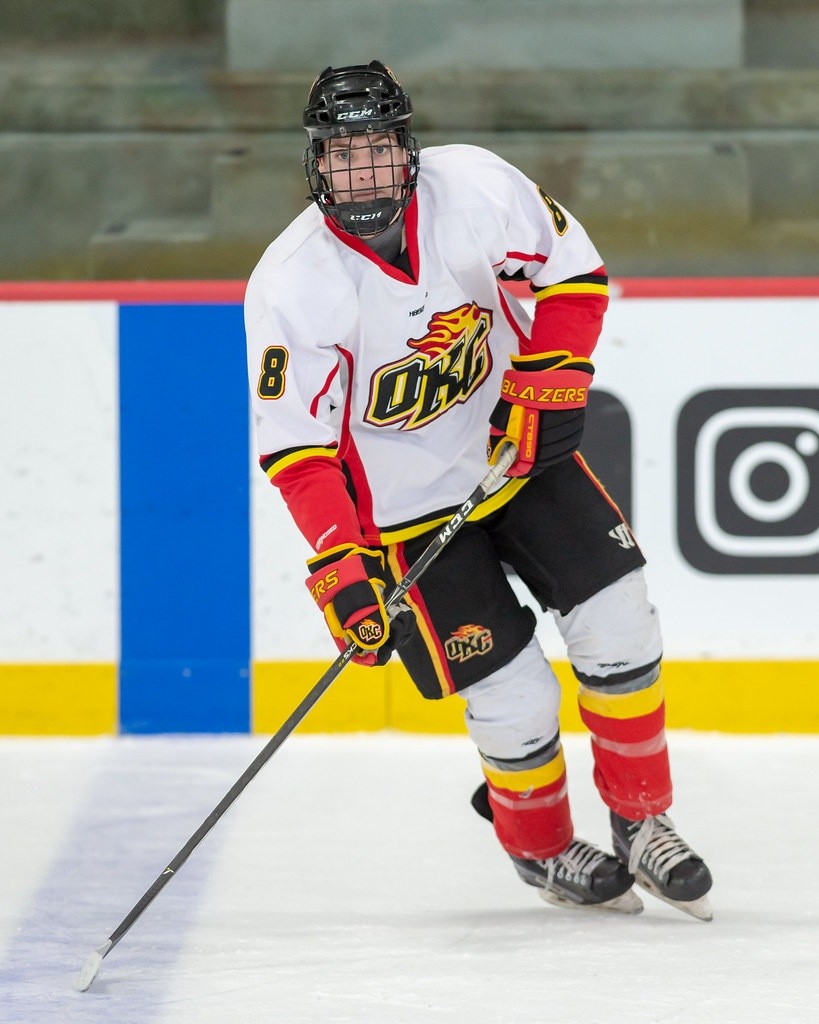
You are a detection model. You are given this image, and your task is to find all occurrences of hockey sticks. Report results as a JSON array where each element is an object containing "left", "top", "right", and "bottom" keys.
[{"left": 72, "top": 443, "right": 520, "bottom": 996}]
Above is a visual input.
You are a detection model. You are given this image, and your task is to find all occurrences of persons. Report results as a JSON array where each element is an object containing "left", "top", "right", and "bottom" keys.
[{"left": 244, "top": 55, "right": 715, "bottom": 926}]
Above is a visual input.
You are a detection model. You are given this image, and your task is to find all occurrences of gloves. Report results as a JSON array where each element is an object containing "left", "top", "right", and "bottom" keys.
[
  {"left": 486, "top": 350, "right": 595, "bottom": 480},
  {"left": 305, "top": 543, "right": 416, "bottom": 667}
]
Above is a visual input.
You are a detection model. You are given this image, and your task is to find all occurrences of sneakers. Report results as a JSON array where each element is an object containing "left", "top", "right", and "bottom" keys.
[
  {"left": 609, "top": 809, "right": 715, "bottom": 923},
  {"left": 471, "top": 781, "right": 644, "bottom": 914}
]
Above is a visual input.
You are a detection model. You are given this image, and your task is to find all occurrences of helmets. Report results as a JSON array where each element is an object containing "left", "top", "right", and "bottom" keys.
[{"left": 302, "top": 60, "right": 420, "bottom": 237}]
